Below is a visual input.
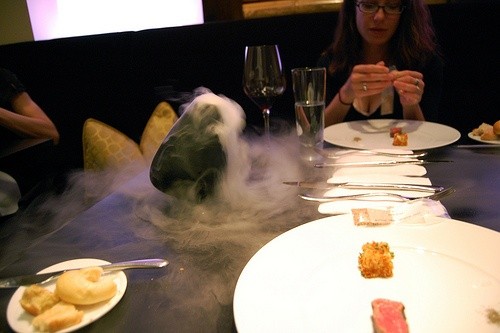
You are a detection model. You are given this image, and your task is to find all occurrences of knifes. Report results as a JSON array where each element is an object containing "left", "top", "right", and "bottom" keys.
[
  {"left": 455, "top": 143, "right": 500, "bottom": 148},
  {"left": 315, "top": 159, "right": 454, "bottom": 169},
  {"left": 0, "top": 258, "right": 170, "bottom": 288},
  {"left": 281, "top": 181, "right": 445, "bottom": 193}
]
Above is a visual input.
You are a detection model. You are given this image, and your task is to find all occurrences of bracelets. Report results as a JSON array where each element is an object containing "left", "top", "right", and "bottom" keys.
[{"left": 338, "top": 91, "right": 352, "bottom": 105}]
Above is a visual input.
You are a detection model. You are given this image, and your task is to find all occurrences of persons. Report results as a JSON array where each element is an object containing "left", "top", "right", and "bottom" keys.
[
  {"left": 315, "top": 0, "right": 451, "bottom": 127},
  {"left": 0, "top": 67, "right": 60, "bottom": 141}
]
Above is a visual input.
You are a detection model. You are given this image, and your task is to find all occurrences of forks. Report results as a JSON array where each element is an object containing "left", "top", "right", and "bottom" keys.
[
  {"left": 312, "top": 146, "right": 427, "bottom": 159},
  {"left": 297, "top": 186, "right": 457, "bottom": 203}
]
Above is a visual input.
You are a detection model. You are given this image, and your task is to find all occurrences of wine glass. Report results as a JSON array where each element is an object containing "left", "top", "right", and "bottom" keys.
[{"left": 243, "top": 43, "right": 286, "bottom": 147}]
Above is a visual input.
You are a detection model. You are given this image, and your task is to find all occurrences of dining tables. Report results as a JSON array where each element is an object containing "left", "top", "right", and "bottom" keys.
[{"left": 0, "top": 127, "right": 500, "bottom": 333}]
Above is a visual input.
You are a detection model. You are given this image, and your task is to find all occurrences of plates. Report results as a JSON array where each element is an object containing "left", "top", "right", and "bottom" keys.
[
  {"left": 467, "top": 131, "right": 500, "bottom": 145},
  {"left": 232, "top": 212, "right": 500, "bottom": 333},
  {"left": 323, "top": 119, "right": 461, "bottom": 151},
  {"left": 6, "top": 258, "right": 128, "bottom": 333}
]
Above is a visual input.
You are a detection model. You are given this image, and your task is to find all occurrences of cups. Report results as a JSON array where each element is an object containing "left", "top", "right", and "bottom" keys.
[{"left": 291, "top": 66, "right": 326, "bottom": 167}]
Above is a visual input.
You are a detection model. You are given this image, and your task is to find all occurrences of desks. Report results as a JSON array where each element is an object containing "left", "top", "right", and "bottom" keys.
[{"left": 0, "top": 135, "right": 55, "bottom": 202}]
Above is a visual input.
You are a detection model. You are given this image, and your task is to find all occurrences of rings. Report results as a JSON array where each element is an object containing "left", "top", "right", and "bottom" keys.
[
  {"left": 415, "top": 79, "right": 419, "bottom": 93},
  {"left": 361, "top": 79, "right": 367, "bottom": 91}
]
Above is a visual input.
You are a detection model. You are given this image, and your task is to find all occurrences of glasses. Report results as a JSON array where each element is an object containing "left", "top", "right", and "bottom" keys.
[{"left": 354, "top": 0, "right": 407, "bottom": 15}]
